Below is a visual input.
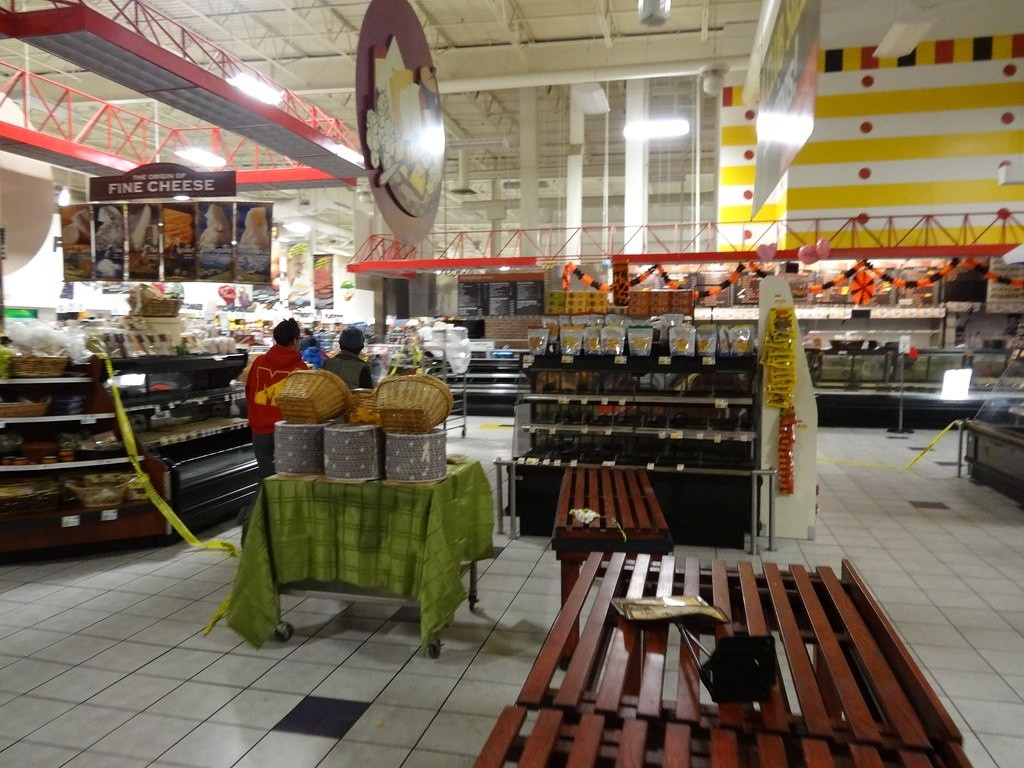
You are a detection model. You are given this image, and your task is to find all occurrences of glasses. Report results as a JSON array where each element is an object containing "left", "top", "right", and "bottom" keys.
[{"left": 296, "top": 337, "right": 303, "bottom": 341}]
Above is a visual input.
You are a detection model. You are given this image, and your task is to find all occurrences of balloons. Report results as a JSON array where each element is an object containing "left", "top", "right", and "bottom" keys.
[
  {"left": 756, "top": 243, "right": 777, "bottom": 263},
  {"left": 816, "top": 239, "right": 829, "bottom": 259},
  {"left": 798, "top": 244, "right": 820, "bottom": 265},
  {"left": 218, "top": 285, "right": 236, "bottom": 305}
]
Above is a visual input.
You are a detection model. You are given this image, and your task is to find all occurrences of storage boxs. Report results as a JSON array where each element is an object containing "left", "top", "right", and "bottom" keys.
[{"left": 544, "top": 287, "right": 694, "bottom": 317}]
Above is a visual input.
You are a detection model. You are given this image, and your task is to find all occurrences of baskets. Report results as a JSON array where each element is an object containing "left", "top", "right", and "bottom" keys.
[
  {"left": 375, "top": 374, "right": 453, "bottom": 435},
  {"left": 275, "top": 369, "right": 353, "bottom": 424},
  {"left": 129, "top": 296, "right": 182, "bottom": 317},
  {"left": 8, "top": 356, "right": 67, "bottom": 377},
  {"left": 344, "top": 388, "right": 379, "bottom": 425},
  {"left": 0, "top": 395, "right": 53, "bottom": 416},
  {"left": 65, "top": 472, "right": 139, "bottom": 506}
]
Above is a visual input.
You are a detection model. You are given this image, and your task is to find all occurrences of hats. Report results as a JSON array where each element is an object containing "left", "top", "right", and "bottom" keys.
[{"left": 338, "top": 326, "right": 365, "bottom": 349}]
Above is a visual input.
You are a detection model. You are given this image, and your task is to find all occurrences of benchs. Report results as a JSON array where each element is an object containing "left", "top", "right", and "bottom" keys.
[
  {"left": 472, "top": 550, "right": 964, "bottom": 749},
  {"left": 472, "top": 706, "right": 974, "bottom": 768},
  {"left": 550, "top": 465, "right": 672, "bottom": 665}
]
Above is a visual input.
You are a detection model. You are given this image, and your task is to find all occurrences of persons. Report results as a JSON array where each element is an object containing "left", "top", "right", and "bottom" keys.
[
  {"left": 280, "top": 243, "right": 311, "bottom": 302},
  {"left": 299, "top": 330, "right": 325, "bottom": 369},
  {"left": 245, "top": 318, "right": 311, "bottom": 484},
  {"left": 324, "top": 326, "right": 374, "bottom": 388}
]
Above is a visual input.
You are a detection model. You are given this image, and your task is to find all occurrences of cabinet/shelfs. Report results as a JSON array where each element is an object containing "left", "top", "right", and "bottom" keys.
[{"left": 0, "top": 322, "right": 1024, "bottom": 559}]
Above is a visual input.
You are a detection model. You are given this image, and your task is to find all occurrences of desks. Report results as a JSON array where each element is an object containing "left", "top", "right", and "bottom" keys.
[{"left": 227, "top": 459, "right": 493, "bottom": 659}]
